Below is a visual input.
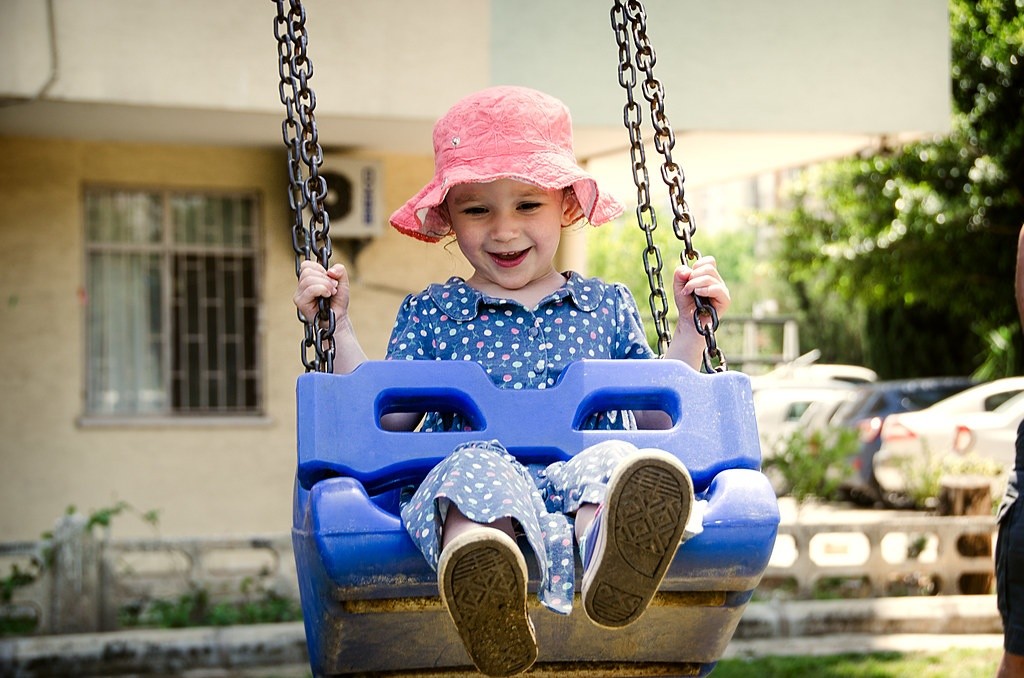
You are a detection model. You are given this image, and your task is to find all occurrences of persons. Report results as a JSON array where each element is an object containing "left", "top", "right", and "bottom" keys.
[
  {"left": 994, "top": 224, "right": 1024, "bottom": 678},
  {"left": 293, "top": 85, "right": 730, "bottom": 678}
]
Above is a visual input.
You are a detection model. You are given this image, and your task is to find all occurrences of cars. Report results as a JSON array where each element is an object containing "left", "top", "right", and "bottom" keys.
[
  {"left": 869, "top": 374, "right": 1024, "bottom": 497},
  {"left": 748, "top": 359, "right": 978, "bottom": 510}
]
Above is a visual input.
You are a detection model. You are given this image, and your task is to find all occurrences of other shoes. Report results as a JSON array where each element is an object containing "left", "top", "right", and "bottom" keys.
[
  {"left": 581, "top": 450, "right": 694, "bottom": 630},
  {"left": 437, "top": 525, "right": 538, "bottom": 677}
]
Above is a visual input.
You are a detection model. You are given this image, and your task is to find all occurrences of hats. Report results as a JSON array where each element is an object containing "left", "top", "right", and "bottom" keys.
[{"left": 390, "top": 86, "right": 625, "bottom": 241}]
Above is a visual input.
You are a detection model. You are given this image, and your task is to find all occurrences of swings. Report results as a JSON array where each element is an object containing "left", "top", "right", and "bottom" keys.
[{"left": 270, "top": 0, "right": 781, "bottom": 678}]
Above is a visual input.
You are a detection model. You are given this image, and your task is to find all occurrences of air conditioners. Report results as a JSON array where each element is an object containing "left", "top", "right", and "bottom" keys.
[{"left": 295, "top": 151, "right": 385, "bottom": 262}]
